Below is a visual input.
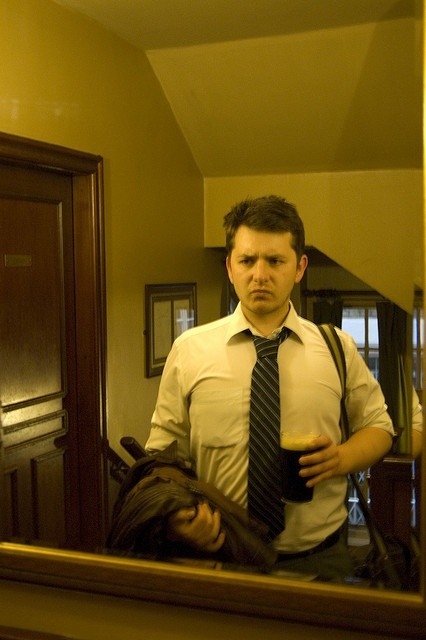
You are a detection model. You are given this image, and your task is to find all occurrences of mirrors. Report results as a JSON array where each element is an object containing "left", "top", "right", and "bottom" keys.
[{"left": 0, "top": 1, "right": 426, "bottom": 633}]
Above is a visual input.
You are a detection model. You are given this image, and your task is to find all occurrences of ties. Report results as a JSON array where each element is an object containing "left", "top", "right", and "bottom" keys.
[{"left": 242, "top": 327, "right": 291, "bottom": 547}]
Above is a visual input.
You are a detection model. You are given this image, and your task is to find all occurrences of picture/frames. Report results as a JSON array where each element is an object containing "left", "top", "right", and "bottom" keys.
[{"left": 146, "top": 284, "right": 197, "bottom": 377}]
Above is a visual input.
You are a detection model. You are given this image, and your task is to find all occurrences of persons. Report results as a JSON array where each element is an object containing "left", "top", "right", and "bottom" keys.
[
  {"left": 143, "top": 196, "right": 394, "bottom": 585},
  {"left": 400, "top": 381, "right": 423, "bottom": 455}
]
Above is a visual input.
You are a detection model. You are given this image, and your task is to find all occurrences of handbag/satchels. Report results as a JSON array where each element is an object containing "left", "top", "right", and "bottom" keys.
[{"left": 341, "top": 533, "right": 420, "bottom": 593}]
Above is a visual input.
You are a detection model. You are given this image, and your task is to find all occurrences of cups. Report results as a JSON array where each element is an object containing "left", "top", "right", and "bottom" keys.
[{"left": 278, "top": 432, "right": 319, "bottom": 504}]
